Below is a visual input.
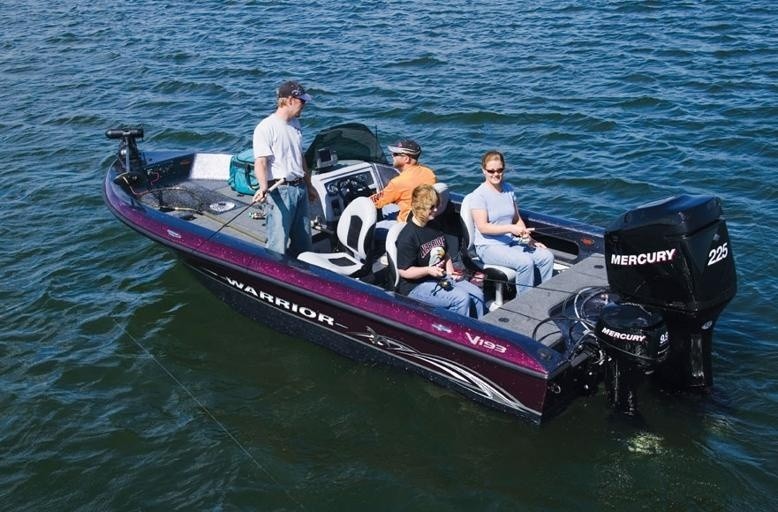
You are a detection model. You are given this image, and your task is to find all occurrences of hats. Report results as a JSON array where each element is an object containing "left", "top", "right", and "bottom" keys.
[
  {"left": 387, "top": 140, "right": 420, "bottom": 157},
  {"left": 279, "top": 81, "right": 311, "bottom": 101}
]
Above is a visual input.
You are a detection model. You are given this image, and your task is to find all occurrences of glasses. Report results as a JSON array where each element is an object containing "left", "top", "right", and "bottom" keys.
[
  {"left": 485, "top": 168, "right": 503, "bottom": 173},
  {"left": 429, "top": 204, "right": 438, "bottom": 210}
]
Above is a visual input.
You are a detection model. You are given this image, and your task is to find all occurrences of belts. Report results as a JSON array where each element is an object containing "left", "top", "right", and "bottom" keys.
[{"left": 267, "top": 178, "right": 304, "bottom": 186}]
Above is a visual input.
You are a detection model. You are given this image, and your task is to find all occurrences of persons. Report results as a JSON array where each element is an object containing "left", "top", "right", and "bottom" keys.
[
  {"left": 472, "top": 151, "right": 554, "bottom": 297},
  {"left": 396, "top": 183, "right": 486, "bottom": 318},
  {"left": 252, "top": 79, "right": 319, "bottom": 254},
  {"left": 368, "top": 139, "right": 437, "bottom": 242}
]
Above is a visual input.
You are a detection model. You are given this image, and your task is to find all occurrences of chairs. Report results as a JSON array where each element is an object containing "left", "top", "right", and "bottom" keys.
[
  {"left": 386, "top": 222, "right": 407, "bottom": 288},
  {"left": 430, "top": 182, "right": 450, "bottom": 214},
  {"left": 297, "top": 196, "right": 377, "bottom": 276},
  {"left": 455, "top": 191, "right": 540, "bottom": 312}
]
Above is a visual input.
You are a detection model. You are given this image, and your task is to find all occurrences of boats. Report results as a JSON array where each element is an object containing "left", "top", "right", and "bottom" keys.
[{"left": 101, "top": 122, "right": 739, "bottom": 432}]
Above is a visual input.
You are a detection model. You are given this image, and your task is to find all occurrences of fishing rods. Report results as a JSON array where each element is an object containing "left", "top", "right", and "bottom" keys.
[
  {"left": 511, "top": 220, "right": 611, "bottom": 245},
  {"left": 111, "top": 177, "right": 286, "bottom": 325},
  {"left": 431, "top": 272, "right": 622, "bottom": 303}
]
